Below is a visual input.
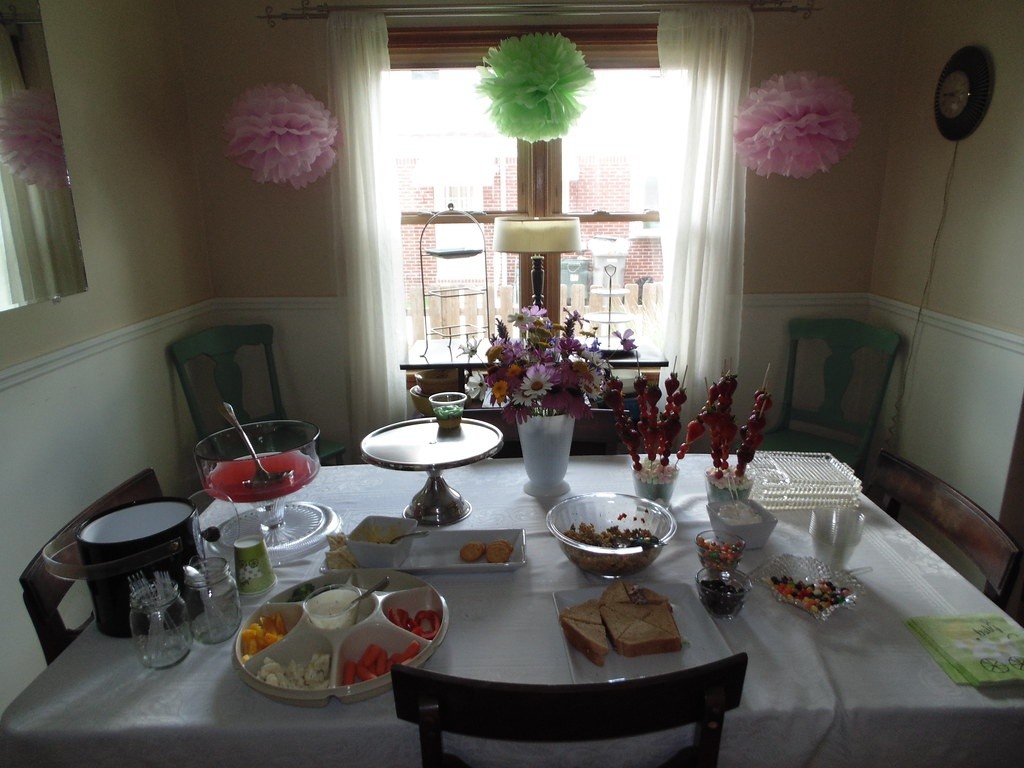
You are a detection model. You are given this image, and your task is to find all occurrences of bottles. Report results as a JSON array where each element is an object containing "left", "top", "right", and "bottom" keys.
[
  {"left": 126, "top": 580, "right": 192, "bottom": 669},
  {"left": 182, "top": 557, "right": 242, "bottom": 644}
]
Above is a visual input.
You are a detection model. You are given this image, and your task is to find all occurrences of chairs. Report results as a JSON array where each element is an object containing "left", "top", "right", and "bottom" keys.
[
  {"left": 170, "top": 323, "right": 348, "bottom": 468},
  {"left": 852, "top": 449, "right": 1024, "bottom": 611},
  {"left": 727, "top": 316, "right": 904, "bottom": 478},
  {"left": 17, "top": 465, "right": 177, "bottom": 664},
  {"left": 392, "top": 652, "right": 748, "bottom": 768}
]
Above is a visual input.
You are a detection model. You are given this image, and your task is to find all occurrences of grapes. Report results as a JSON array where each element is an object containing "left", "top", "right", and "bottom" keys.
[{"left": 613, "top": 393, "right": 765, "bottom": 479}]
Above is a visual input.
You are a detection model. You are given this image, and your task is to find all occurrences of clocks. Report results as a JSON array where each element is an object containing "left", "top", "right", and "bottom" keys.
[{"left": 934, "top": 44, "right": 995, "bottom": 140}]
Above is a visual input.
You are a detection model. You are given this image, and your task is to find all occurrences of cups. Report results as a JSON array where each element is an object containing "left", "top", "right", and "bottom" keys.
[
  {"left": 428, "top": 391, "right": 468, "bottom": 430},
  {"left": 231, "top": 535, "right": 277, "bottom": 598},
  {"left": 631, "top": 461, "right": 678, "bottom": 507},
  {"left": 705, "top": 466, "right": 756, "bottom": 504}
]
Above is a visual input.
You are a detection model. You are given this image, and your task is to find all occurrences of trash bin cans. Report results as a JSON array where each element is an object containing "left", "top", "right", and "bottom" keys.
[{"left": 562, "top": 256, "right": 590, "bottom": 303}]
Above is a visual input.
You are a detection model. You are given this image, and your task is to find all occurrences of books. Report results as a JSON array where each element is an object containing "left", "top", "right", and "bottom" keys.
[{"left": 906, "top": 615, "right": 1024, "bottom": 688}]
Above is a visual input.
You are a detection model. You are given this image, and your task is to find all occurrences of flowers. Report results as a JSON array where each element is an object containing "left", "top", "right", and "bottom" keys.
[{"left": 461, "top": 303, "right": 616, "bottom": 424}]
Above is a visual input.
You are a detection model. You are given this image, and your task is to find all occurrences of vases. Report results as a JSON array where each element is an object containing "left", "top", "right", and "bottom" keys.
[{"left": 515, "top": 406, "right": 576, "bottom": 498}]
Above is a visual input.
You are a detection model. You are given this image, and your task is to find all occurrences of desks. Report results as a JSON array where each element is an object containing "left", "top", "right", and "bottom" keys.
[{"left": 0, "top": 451, "right": 1024, "bottom": 768}]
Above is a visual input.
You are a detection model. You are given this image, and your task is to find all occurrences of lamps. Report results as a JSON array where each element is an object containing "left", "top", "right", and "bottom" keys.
[{"left": 492, "top": 216, "right": 585, "bottom": 308}]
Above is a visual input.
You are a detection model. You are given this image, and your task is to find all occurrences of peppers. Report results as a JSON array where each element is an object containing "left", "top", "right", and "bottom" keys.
[
  {"left": 242, "top": 611, "right": 289, "bottom": 663},
  {"left": 389, "top": 608, "right": 441, "bottom": 641}
]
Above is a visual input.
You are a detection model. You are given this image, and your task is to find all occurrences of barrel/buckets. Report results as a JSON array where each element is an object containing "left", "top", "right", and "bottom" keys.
[{"left": 39, "top": 494, "right": 210, "bottom": 639}]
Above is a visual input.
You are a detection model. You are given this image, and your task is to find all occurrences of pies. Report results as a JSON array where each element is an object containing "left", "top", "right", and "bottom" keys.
[
  {"left": 324, "top": 532, "right": 362, "bottom": 570},
  {"left": 460, "top": 538, "right": 514, "bottom": 563}
]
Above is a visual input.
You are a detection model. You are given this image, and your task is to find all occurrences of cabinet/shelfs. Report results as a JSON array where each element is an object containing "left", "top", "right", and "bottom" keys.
[{"left": 397, "top": 334, "right": 669, "bottom": 456}]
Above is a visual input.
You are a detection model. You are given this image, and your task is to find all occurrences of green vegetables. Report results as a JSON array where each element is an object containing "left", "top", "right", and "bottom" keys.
[{"left": 286, "top": 583, "right": 318, "bottom": 603}]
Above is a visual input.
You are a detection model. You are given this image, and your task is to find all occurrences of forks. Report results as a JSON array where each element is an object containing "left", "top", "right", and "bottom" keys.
[{"left": 126, "top": 567, "right": 188, "bottom": 663}]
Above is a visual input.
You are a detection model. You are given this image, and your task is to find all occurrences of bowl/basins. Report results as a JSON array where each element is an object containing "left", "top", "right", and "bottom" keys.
[
  {"left": 411, "top": 368, "right": 467, "bottom": 416},
  {"left": 345, "top": 516, "right": 418, "bottom": 569},
  {"left": 695, "top": 529, "right": 747, "bottom": 573},
  {"left": 695, "top": 566, "right": 753, "bottom": 618},
  {"left": 304, "top": 582, "right": 362, "bottom": 629},
  {"left": 705, "top": 499, "right": 779, "bottom": 553},
  {"left": 545, "top": 491, "right": 677, "bottom": 576}
]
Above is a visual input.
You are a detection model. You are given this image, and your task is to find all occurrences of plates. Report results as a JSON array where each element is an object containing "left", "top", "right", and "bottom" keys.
[
  {"left": 318, "top": 526, "right": 526, "bottom": 580},
  {"left": 554, "top": 579, "right": 733, "bottom": 687},
  {"left": 757, "top": 555, "right": 863, "bottom": 619}
]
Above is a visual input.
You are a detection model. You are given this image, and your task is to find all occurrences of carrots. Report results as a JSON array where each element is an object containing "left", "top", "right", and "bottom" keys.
[{"left": 342, "top": 640, "right": 420, "bottom": 687}]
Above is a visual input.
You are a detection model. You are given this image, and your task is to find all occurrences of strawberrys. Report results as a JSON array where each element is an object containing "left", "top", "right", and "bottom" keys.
[{"left": 601, "top": 373, "right": 773, "bottom": 453}]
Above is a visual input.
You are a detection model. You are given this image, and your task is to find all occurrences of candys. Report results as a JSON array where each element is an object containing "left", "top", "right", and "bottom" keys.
[
  {"left": 765, "top": 574, "right": 849, "bottom": 614},
  {"left": 697, "top": 536, "right": 743, "bottom": 569}
]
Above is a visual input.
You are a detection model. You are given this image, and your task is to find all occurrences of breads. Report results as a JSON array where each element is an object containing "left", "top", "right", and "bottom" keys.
[{"left": 560, "top": 580, "right": 682, "bottom": 668}]
